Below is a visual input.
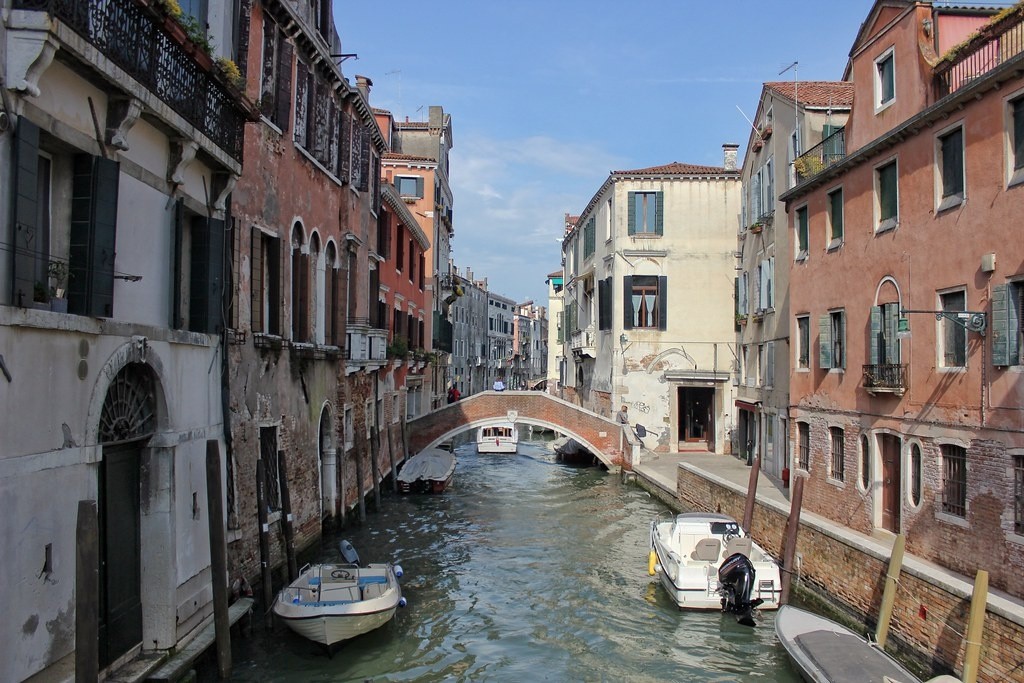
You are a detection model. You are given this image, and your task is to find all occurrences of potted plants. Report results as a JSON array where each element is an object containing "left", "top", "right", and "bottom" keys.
[
  {"left": 761, "top": 125, "right": 772, "bottom": 140},
  {"left": 736, "top": 314, "right": 748, "bottom": 325},
  {"left": 748, "top": 222, "right": 764, "bottom": 235},
  {"left": 752, "top": 139, "right": 762, "bottom": 153}
]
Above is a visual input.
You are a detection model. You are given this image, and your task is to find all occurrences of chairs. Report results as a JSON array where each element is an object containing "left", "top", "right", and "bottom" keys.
[
  {"left": 724, "top": 538, "right": 752, "bottom": 561},
  {"left": 697, "top": 540, "right": 721, "bottom": 560}
]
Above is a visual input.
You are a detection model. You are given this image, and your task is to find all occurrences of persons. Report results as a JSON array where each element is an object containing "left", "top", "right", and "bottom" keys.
[
  {"left": 447, "top": 384, "right": 461, "bottom": 404},
  {"left": 616, "top": 405, "right": 630, "bottom": 424},
  {"left": 493, "top": 376, "right": 505, "bottom": 392}
]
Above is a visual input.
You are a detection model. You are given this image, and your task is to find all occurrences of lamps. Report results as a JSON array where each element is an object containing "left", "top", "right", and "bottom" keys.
[
  {"left": 896, "top": 305, "right": 987, "bottom": 340},
  {"left": 34, "top": 260, "right": 71, "bottom": 304}
]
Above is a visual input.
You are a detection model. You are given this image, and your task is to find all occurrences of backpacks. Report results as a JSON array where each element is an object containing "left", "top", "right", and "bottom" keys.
[{"left": 447, "top": 392, "right": 455, "bottom": 404}]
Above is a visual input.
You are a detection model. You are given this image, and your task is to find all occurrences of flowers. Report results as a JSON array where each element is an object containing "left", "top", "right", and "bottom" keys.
[{"left": 159, "top": 0, "right": 240, "bottom": 81}]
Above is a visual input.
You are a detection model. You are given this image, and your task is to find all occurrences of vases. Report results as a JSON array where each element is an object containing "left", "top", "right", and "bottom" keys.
[{"left": 141, "top": 0, "right": 228, "bottom": 85}]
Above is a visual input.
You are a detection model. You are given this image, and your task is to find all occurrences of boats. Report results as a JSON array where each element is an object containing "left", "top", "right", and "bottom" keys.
[
  {"left": 272, "top": 540, "right": 407, "bottom": 661},
  {"left": 397, "top": 447, "right": 458, "bottom": 493},
  {"left": 773, "top": 603, "right": 963, "bottom": 683},
  {"left": 647, "top": 508, "right": 783, "bottom": 628},
  {"left": 476, "top": 423, "right": 520, "bottom": 453}
]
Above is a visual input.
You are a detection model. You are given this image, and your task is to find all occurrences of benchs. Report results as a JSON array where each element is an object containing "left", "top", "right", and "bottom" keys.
[{"left": 309, "top": 568, "right": 387, "bottom": 600}]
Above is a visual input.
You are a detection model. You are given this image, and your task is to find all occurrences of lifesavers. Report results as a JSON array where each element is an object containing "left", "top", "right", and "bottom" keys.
[{"left": 233, "top": 577, "right": 253, "bottom": 600}]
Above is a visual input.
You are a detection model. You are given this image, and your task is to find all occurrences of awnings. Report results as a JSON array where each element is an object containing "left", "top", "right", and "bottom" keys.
[{"left": 552, "top": 271, "right": 590, "bottom": 291}]
